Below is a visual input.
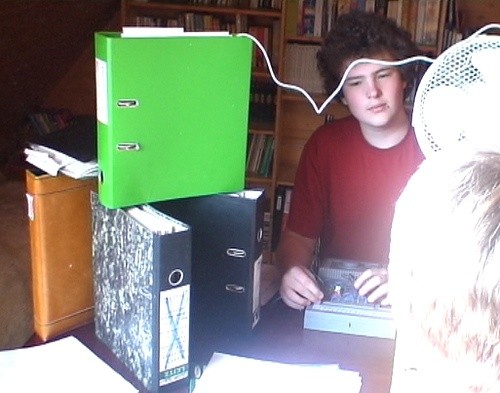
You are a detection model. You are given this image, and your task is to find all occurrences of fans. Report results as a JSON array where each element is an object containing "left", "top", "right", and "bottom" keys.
[{"left": 411, "top": 34, "right": 500, "bottom": 158}]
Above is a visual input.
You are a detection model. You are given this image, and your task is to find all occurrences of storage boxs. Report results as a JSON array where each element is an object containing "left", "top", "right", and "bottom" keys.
[{"left": 304, "top": 268, "right": 396, "bottom": 341}]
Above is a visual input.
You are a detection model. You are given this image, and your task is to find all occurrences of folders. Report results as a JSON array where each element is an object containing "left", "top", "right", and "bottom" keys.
[
  {"left": 89, "top": 187, "right": 194, "bottom": 393},
  {"left": 93, "top": 29, "right": 253, "bottom": 212},
  {"left": 164, "top": 189, "right": 271, "bottom": 338}
]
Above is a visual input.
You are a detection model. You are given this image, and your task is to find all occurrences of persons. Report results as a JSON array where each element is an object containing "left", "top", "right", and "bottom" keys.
[{"left": 280, "top": 9, "right": 427, "bottom": 308}]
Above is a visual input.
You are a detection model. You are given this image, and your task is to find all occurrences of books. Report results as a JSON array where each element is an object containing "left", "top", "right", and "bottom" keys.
[{"left": 123, "top": 0, "right": 487, "bottom": 263}]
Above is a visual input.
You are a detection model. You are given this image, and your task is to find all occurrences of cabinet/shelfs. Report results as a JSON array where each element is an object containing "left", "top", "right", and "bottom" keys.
[
  {"left": 120, "top": 0, "right": 287, "bottom": 265},
  {"left": 272, "top": 0, "right": 448, "bottom": 262}
]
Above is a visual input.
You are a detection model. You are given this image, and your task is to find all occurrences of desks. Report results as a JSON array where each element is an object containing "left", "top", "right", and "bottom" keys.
[{"left": 23, "top": 291, "right": 396, "bottom": 393}]
